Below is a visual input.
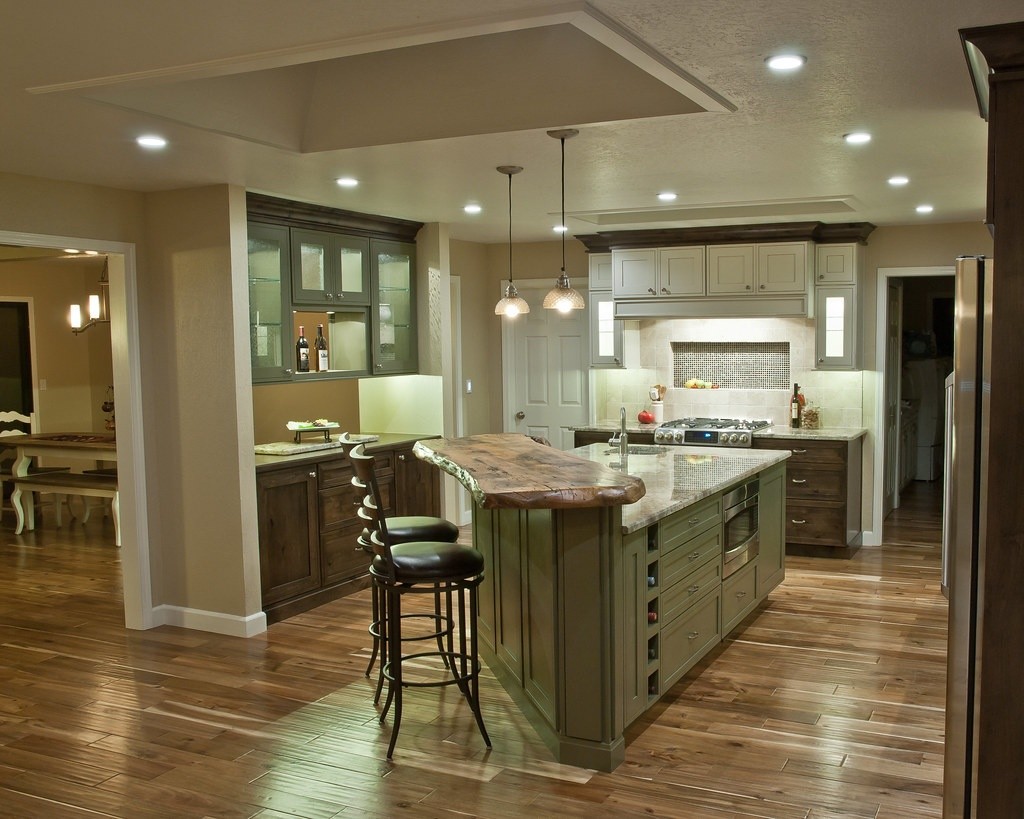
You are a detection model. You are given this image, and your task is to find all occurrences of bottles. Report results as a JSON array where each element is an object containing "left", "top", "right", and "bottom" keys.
[
  {"left": 314, "top": 324, "right": 329, "bottom": 372},
  {"left": 791, "top": 382, "right": 800, "bottom": 428},
  {"left": 296, "top": 326, "right": 310, "bottom": 372},
  {"left": 801, "top": 401, "right": 820, "bottom": 430}
]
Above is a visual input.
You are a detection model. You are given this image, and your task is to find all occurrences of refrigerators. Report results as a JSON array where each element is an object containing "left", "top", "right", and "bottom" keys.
[{"left": 939, "top": 254, "right": 995, "bottom": 819}]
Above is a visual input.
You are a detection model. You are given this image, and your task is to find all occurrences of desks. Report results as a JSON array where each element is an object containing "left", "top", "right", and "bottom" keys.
[{"left": 0, "top": 431, "right": 116, "bottom": 534}]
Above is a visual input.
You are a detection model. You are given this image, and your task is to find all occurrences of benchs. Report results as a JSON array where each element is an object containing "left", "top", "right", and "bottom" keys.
[{"left": 0, "top": 466, "right": 121, "bottom": 547}]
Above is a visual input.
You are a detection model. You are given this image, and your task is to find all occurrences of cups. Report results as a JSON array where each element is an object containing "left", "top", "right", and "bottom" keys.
[{"left": 650, "top": 401, "right": 663, "bottom": 423}]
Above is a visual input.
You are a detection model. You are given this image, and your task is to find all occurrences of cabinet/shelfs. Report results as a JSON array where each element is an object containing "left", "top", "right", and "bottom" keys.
[
  {"left": 589, "top": 241, "right": 862, "bottom": 372},
  {"left": 255, "top": 442, "right": 444, "bottom": 625},
  {"left": 901, "top": 409, "right": 917, "bottom": 492},
  {"left": 246, "top": 221, "right": 419, "bottom": 385},
  {"left": 575, "top": 430, "right": 849, "bottom": 726}
]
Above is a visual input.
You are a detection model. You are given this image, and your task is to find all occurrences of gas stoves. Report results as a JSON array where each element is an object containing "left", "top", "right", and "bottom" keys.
[{"left": 653, "top": 416, "right": 773, "bottom": 447}]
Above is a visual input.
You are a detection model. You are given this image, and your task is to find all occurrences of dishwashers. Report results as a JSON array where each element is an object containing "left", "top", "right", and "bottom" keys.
[{"left": 722, "top": 473, "right": 760, "bottom": 642}]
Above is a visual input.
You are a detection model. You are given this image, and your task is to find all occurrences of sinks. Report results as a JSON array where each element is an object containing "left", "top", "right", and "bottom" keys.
[{"left": 603, "top": 446, "right": 674, "bottom": 455}]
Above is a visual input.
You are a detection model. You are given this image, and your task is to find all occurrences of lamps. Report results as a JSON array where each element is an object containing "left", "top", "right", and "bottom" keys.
[
  {"left": 70, "top": 254, "right": 111, "bottom": 335},
  {"left": 494, "top": 166, "right": 530, "bottom": 316},
  {"left": 543, "top": 128, "right": 585, "bottom": 314}
]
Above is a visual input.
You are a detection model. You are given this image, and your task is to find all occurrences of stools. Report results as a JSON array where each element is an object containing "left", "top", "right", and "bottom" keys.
[
  {"left": 349, "top": 445, "right": 492, "bottom": 761},
  {"left": 335, "top": 432, "right": 465, "bottom": 708}
]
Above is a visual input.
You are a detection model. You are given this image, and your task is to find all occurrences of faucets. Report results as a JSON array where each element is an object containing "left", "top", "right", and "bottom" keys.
[{"left": 608, "top": 407, "right": 628, "bottom": 456}]
